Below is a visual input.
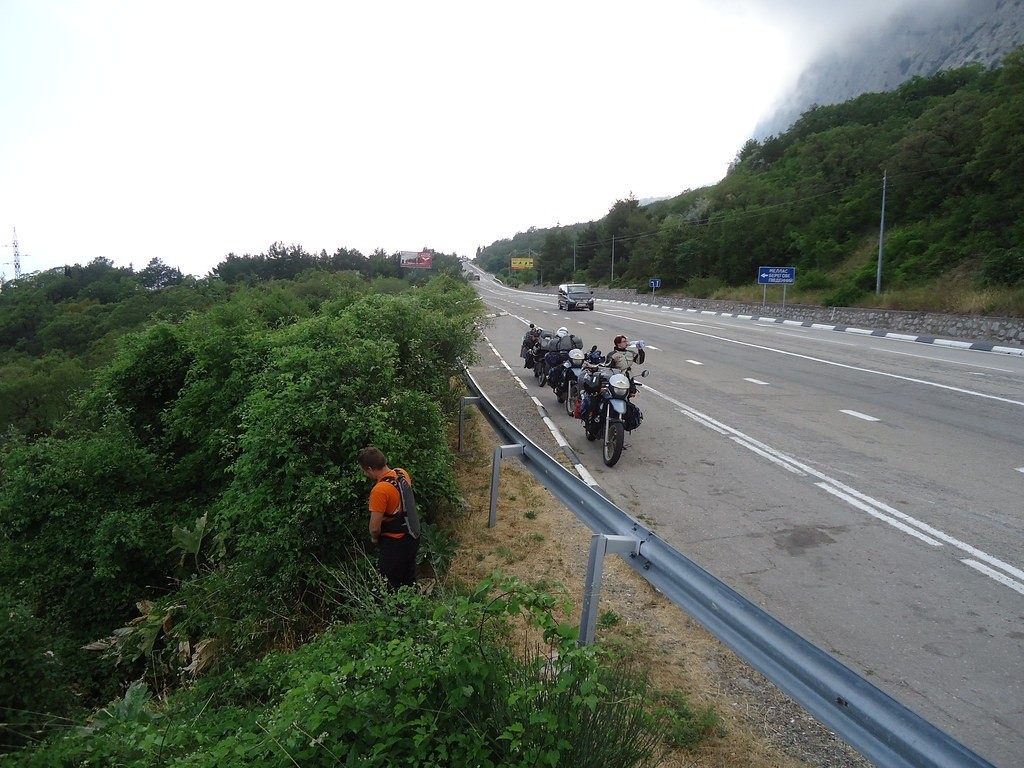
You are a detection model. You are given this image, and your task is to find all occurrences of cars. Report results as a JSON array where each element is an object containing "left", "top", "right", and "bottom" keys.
[{"left": 458, "top": 256, "right": 481, "bottom": 281}]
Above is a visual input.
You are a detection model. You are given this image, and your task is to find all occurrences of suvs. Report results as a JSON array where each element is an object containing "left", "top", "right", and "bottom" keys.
[{"left": 557, "top": 284, "right": 594, "bottom": 312}]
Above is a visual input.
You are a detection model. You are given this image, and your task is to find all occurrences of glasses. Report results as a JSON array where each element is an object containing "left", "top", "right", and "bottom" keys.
[{"left": 621, "top": 341, "right": 628, "bottom": 343}]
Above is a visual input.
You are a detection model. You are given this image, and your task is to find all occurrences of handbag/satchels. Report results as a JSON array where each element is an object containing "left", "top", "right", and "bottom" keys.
[
  {"left": 580, "top": 395, "right": 597, "bottom": 421},
  {"left": 573, "top": 399, "right": 585, "bottom": 419},
  {"left": 524, "top": 349, "right": 535, "bottom": 369},
  {"left": 520, "top": 345, "right": 527, "bottom": 358},
  {"left": 622, "top": 401, "right": 643, "bottom": 432},
  {"left": 547, "top": 365, "right": 561, "bottom": 389}
]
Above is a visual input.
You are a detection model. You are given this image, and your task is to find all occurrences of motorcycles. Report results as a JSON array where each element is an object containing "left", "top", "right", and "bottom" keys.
[{"left": 518, "top": 322, "right": 649, "bottom": 468}]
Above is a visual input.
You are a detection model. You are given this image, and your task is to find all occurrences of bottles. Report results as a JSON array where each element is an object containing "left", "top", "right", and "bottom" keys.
[{"left": 626, "top": 340, "right": 645, "bottom": 348}]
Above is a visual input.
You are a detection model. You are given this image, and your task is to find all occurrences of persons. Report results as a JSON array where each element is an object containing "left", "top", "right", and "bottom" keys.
[
  {"left": 606, "top": 335, "right": 645, "bottom": 383},
  {"left": 357, "top": 446, "right": 421, "bottom": 599}
]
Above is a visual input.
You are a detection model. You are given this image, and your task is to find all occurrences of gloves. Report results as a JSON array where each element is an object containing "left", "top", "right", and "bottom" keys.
[
  {"left": 583, "top": 361, "right": 592, "bottom": 368},
  {"left": 636, "top": 343, "right": 641, "bottom": 349}
]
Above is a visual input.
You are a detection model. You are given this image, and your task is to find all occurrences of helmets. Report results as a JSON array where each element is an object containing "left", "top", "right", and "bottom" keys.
[
  {"left": 591, "top": 350, "right": 606, "bottom": 363},
  {"left": 557, "top": 327, "right": 569, "bottom": 337},
  {"left": 583, "top": 375, "right": 601, "bottom": 393},
  {"left": 545, "top": 352, "right": 562, "bottom": 366}
]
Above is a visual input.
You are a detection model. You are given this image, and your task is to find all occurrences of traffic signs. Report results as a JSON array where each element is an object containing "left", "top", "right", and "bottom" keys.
[{"left": 758, "top": 266, "right": 797, "bottom": 286}]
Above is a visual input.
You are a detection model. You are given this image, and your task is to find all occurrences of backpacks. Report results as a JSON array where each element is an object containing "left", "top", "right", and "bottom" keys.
[{"left": 380, "top": 469, "right": 420, "bottom": 539}]
